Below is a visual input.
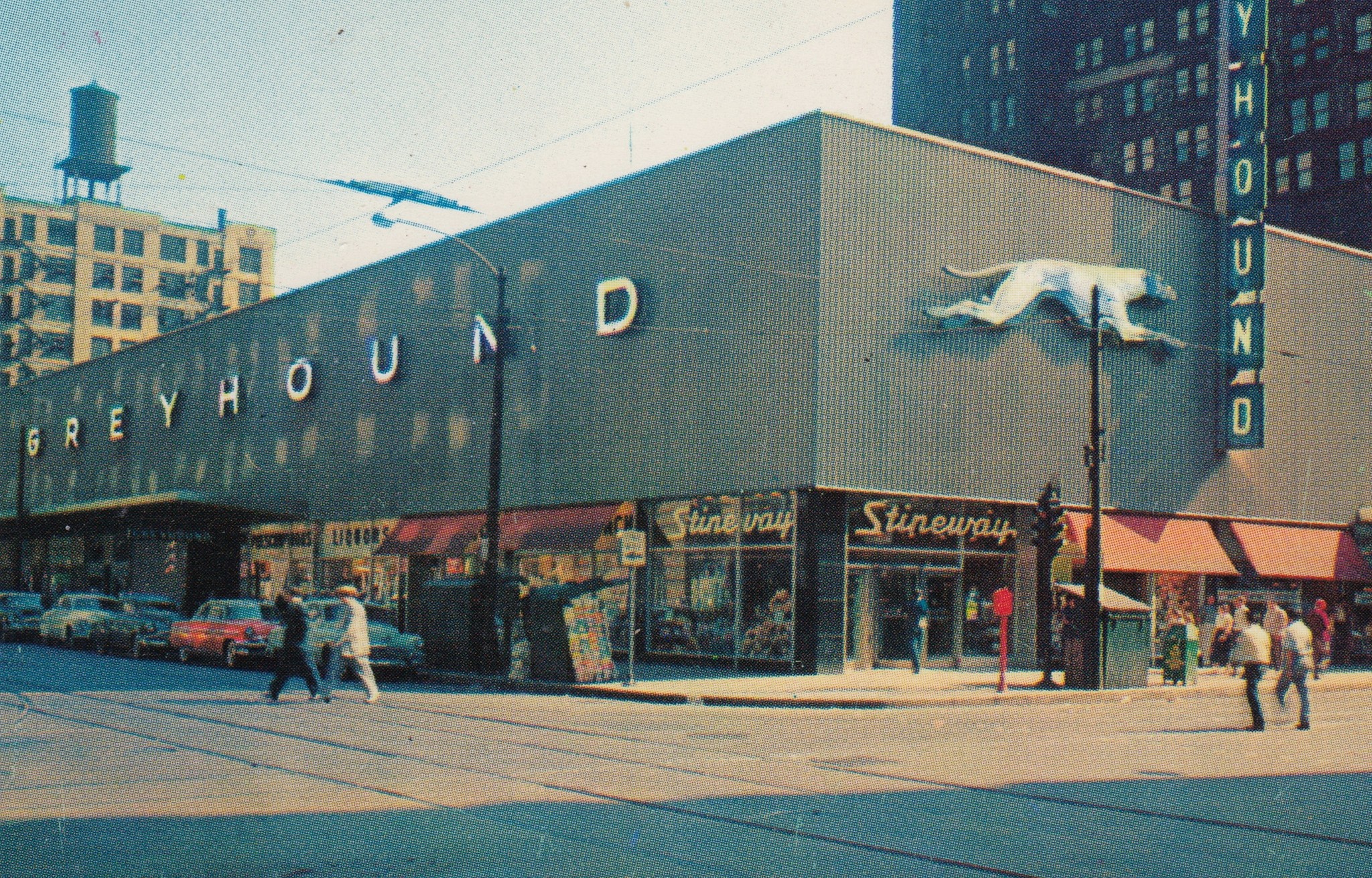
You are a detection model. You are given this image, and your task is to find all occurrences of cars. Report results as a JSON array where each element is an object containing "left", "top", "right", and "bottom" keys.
[
  {"left": 39, "top": 593, "right": 119, "bottom": 648},
  {"left": 267, "top": 599, "right": 426, "bottom": 680},
  {"left": 92, "top": 592, "right": 187, "bottom": 658},
  {"left": 0, "top": 592, "right": 47, "bottom": 641},
  {"left": 170, "top": 600, "right": 281, "bottom": 670}
]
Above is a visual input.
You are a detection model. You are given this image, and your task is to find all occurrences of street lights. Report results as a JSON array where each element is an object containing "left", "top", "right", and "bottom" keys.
[{"left": 371, "top": 211, "right": 511, "bottom": 670}]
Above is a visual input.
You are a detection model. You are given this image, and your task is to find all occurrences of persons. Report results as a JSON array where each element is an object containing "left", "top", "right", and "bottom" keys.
[
  {"left": 903, "top": 588, "right": 932, "bottom": 673},
  {"left": 1167, "top": 595, "right": 1332, "bottom": 731},
  {"left": 319, "top": 585, "right": 379, "bottom": 704},
  {"left": 260, "top": 587, "right": 322, "bottom": 700},
  {"left": 1057, "top": 594, "right": 1076, "bottom": 665}
]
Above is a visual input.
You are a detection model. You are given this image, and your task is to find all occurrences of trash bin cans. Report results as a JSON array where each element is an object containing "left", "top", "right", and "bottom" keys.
[
  {"left": 520, "top": 579, "right": 619, "bottom": 682},
  {"left": 1052, "top": 584, "right": 1153, "bottom": 691},
  {"left": 1159, "top": 621, "right": 1200, "bottom": 687},
  {"left": 421, "top": 573, "right": 530, "bottom": 676}
]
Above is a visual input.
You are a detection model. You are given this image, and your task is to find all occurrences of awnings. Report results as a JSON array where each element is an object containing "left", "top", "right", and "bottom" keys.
[
  {"left": 1064, "top": 512, "right": 1241, "bottom": 577},
  {"left": 1, "top": 489, "right": 283, "bottom": 530},
  {"left": 1229, "top": 521, "right": 1372, "bottom": 584}
]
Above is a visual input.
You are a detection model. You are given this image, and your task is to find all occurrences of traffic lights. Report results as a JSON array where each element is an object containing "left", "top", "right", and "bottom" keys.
[{"left": 1029, "top": 490, "right": 1067, "bottom": 560}]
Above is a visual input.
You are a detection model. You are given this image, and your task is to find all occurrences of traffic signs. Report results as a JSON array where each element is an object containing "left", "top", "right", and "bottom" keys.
[{"left": 616, "top": 529, "right": 650, "bottom": 568}]
